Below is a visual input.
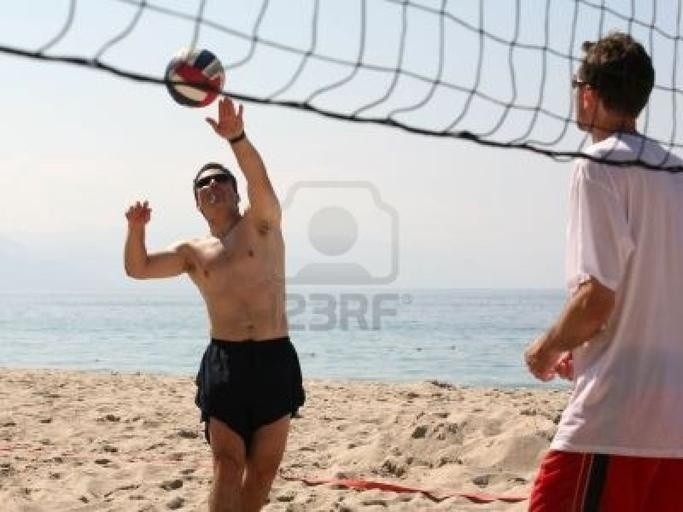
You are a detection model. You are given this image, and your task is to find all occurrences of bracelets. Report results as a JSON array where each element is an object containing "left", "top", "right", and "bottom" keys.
[{"left": 226, "top": 131, "right": 245, "bottom": 144}]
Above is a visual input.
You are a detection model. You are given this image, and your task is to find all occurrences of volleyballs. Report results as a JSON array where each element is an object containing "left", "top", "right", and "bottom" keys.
[{"left": 165, "top": 49, "right": 224, "bottom": 107}]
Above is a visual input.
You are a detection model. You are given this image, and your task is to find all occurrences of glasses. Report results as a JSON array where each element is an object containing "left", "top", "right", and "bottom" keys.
[
  {"left": 194, "top": 172, "right": 232, "bottom": 184},
  {"left": 571, "top": 74, "right": 588, "bottom": 89}
]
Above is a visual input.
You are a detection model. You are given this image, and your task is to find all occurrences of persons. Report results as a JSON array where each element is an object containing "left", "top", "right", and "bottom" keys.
[
  {"left": 120, "top": 93, "right": 305, "bottom": 510},
  {"left": 523, "top": 31, "right": 681, "bottom": 511}
]
